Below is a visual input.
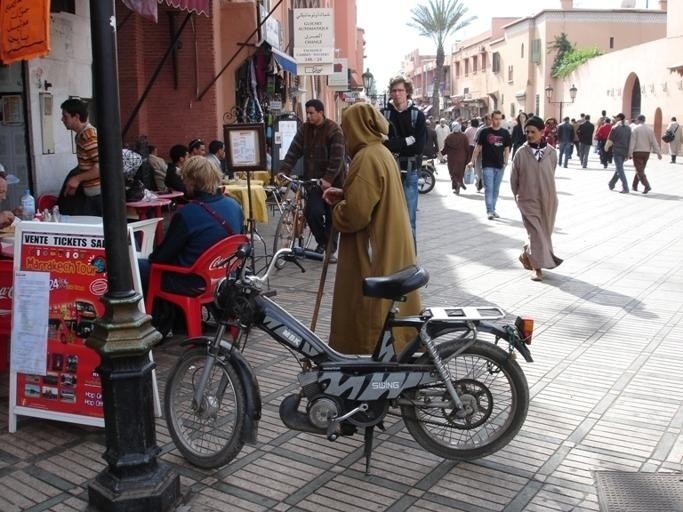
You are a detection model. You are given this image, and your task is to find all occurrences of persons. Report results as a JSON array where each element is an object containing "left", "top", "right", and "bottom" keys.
[
  {"left": 138, "top": 138, "right": 228, "bottom": 195},
  {"left": 380, "top": 73, "right": 427, "bottom": 261},
  {"left": 277, "top": 98, "right": 350, "bottom": 263},
  {"left": 137, "top": 155, "right": 245, "bottom": 340},
  {"left": 322, "top": 101, "right": 429, "bottom": 353},
  {"left": 510, "top": 117, "right": 564, "bottom": 282},
  {"left": 59, "top": 96, "right": 102, "bottom": 214},
  {"left": 426, "top": 109, "right": 682, "bottom": 219},
  {"left": 1, "top": 169, "right": 14, "bottom": 228}
]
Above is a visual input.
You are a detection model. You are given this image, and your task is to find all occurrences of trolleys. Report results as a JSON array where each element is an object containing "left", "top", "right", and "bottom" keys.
[{"left": 163, "top": 242, "right": 534, "bottom": 476}]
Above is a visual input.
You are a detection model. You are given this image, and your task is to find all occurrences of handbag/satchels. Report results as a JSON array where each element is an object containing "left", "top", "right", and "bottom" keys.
[{"left": 662, "top": 131, "right": 675, "bottom": 143}]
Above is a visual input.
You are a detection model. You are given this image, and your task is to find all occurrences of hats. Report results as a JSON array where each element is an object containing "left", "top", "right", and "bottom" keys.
[
  {"left": 524, "top": 116, "right": 544, "bottom": 129},
  {"left": 0, "top": 164, "right": 20, "bottom": 185}
]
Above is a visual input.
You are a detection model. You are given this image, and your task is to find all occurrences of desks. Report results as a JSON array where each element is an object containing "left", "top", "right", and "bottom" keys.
[
  {"left": 225, "top": 178, "right": 263, "bottom": 183},
  {"left": 222, "top": 184, "right": 268, "bottom": 224},
  {"left": 235, "top": 171, "right": 269, "bottom": 182},
  {"left": 129, "top": 199, "right": 171, "bottom": 246},
  {"left": 157, "top": 189, "right": 183, "bottom": 198}
]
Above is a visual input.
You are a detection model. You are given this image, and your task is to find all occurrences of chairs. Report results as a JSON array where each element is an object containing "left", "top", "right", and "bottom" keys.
[
  {"left": 264, "top": 175, "right": 294, "bottom": 215},
  {"left": 37, "top": 192, "right": 58, "bottom": 213},
  {"left": 146, "top": 234, "right": 248, "bottom": 349},
  {"left": 129, "top": 217, "right": 163, "bottom": 259}
]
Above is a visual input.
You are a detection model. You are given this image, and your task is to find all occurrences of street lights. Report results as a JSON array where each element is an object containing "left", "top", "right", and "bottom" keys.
[
  {"left": 544, "top": 82, "right": 578, "bottom": 124},
  {"left": 361, "top": 66, "right": 386, "bottom": 113}
]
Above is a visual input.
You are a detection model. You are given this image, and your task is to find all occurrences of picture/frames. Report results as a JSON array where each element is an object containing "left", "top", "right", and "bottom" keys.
[{"left": 223, "top": 123, "right": 267, "bottom": 171}]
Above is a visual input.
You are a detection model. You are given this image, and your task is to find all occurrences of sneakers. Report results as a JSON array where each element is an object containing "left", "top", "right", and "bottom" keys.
[
  {"left": 487, "top": 213, "right": 494, "bottom": 220},
  {"left": 608, "top": 183, "right": 651, "bottom": 193},
  {"left": 494, "top": 213, "right": 499, "bottom": 218},
  {"left": 315, "top": 245, "right": 324, "bottom": 254},
  {"left": 329, "top": 255, "right": 337, "bottom": 263},
  {"left": 519, "top": 254, "right": 533, "bottom": 270},
  {"left": 531, "top": 270, "right": 543, "bottom": 280}
]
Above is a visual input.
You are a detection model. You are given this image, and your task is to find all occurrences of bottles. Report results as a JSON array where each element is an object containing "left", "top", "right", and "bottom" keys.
[
  {"left": 35, "top": 209, "right": 42, "bottom": 222},
  {"left": 21, "top": 188, "right": 35, "bottom": 221}
]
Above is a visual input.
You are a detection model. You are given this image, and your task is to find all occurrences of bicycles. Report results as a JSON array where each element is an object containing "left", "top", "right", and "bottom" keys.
[
  {"left": 268, "top": 172, "right": 313, "bottom": 271},
  {"left": 415, "top": 168, "right": 434, "bottom": 194}
]
